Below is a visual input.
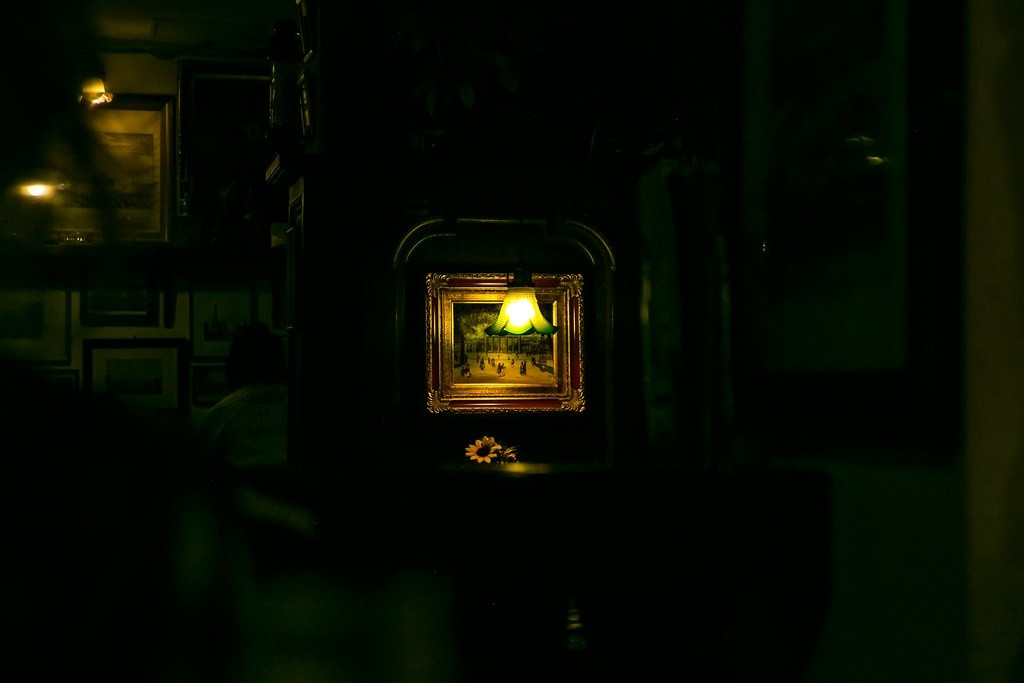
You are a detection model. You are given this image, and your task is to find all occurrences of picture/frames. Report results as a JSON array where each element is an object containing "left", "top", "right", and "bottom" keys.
[
  {"left": 32, "top": 91, "right": 173, "bottom": 244},
  {"left": 424, "top": 271, "right": 592, "bottom": 417},
  {"left": 80, "top": 262, "right": 161, "bottom": 329},
  {"left": 704, "top": 0, "right": 965, "bottom": 463},
  {"left": 187, "top": 272, "right": 280, "bottom": 406},
  {"left": 174, "top": 56, "right": 272, "bottom": 226},
  {"left": 0, "top": 276, "right": 71, "bottom": 368},
  {"left": 81, "top": 334, "right": 188, "bottom": 411}
]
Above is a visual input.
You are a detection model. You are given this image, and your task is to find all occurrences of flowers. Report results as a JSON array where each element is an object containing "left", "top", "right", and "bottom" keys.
[{"left": 464, "top": 433, "right": 519, "bottom": 467}]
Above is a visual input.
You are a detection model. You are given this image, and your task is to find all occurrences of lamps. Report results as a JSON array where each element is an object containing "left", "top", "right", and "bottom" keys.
[
  {"left": 485, "top": 85, "right": 562, "bottom": 337},
  {"left": 81, "top": 51, "right": 116, "bottom": 111}
]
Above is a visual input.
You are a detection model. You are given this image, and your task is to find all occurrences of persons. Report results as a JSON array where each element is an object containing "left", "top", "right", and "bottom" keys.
[{"left": 198, "top": 321, "right": 288, "bottom": 468}]
